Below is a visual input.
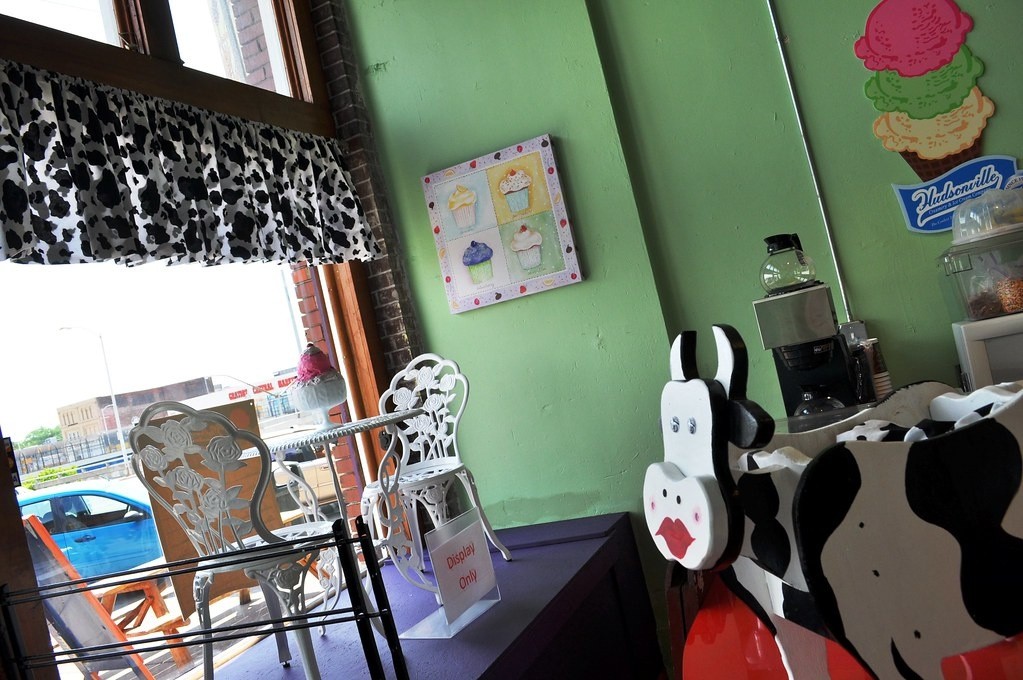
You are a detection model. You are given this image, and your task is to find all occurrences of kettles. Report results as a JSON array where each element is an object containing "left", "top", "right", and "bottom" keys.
[{"left": 758, "top": 232, "right": 817, "bottom": 295}]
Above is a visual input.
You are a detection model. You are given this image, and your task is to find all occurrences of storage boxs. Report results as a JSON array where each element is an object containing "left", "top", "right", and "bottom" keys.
[{"left": 935, "top": 229, "right": 1023, "bottom": 323}]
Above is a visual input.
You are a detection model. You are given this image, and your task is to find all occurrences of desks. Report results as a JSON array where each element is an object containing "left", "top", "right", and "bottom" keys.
[
  {"left": 240, "top": 408, "right": 428, "bottom": 636},
  {"left": 85, "top": 508, "right": 331, "bottom": 678}
]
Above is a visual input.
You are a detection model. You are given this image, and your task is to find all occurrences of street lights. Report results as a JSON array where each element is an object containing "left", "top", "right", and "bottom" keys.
[{"left": 55, "top": 326, "right": 133, "bottom": 478}]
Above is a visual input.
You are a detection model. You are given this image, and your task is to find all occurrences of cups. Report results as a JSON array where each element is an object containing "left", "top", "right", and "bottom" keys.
[{"left": 860, "top": 338, "right": 893, "bottom": 400}]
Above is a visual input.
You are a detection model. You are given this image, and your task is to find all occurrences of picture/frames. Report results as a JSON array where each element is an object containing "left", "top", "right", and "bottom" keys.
[{"left": 421, "top": 133, "right": 584, "bottom": 316}]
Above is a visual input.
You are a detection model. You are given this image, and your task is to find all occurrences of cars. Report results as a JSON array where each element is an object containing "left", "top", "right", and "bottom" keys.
[
  {"left": 239, "top": 424, "right": 337, "bottom": 513},
  {"left": 18, "top": 488, "right": 168, "bottom": 599}
]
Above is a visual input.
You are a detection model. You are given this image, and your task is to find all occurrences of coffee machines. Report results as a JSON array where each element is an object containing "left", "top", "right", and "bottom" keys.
[{"left": 752, "top": 280, "right": 857, "bottom": 417}]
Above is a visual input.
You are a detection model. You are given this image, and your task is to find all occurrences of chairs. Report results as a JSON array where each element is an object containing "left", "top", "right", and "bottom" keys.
[
  {"left": 360, "top": 353, "right": 513, "bottom": 606},
  {"left": 130, "top": 401, "right": 387, "bottom": 680}
]
